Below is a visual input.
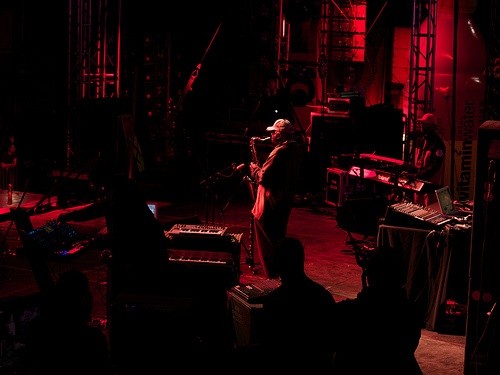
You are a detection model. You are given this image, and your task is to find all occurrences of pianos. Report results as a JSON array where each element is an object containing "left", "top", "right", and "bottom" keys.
[
  {"left": 348, "top": 153, "right": 445, "bottom": 218},
  {"left": 164, "top": 223, "right": 243, "bottom": 301}
]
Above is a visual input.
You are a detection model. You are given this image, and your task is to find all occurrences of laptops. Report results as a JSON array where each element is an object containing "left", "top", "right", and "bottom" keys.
[{"left": 434, "top": 185, "right": 471, "bottom": 218}]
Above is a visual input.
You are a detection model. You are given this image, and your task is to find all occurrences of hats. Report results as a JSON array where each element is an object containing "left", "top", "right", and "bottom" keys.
[
  {"left": 266, "top": 119, "right": 294, "bottom": 132},
  {"left": 417, "top": 113, "right": 437, "bottom": 125}
]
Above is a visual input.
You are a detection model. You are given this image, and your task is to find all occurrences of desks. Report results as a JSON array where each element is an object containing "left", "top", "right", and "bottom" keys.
[{"left": 377, "top": 201, "right": 472, "bottom": 332}]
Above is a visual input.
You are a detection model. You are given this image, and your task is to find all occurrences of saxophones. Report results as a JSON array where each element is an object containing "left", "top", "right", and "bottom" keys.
[{"left": 243, "top": 136, "right": 271, "bottom": 202}]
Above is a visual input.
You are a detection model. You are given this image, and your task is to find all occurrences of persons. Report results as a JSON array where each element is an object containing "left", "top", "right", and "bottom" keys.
[
  {"left": 24, "top": 236, "right": 428, "bottom": 374},
  {"left": 236, "top": 118, "right": 304, "bottom": 279},
  {"left": 412, "top": 111, "right": 446, "bottom": 206}
]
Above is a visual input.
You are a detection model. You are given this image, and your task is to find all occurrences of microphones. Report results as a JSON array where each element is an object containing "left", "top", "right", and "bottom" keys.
[{"left": 236, "top": 163, "right": 246, "bottom": 170}]
